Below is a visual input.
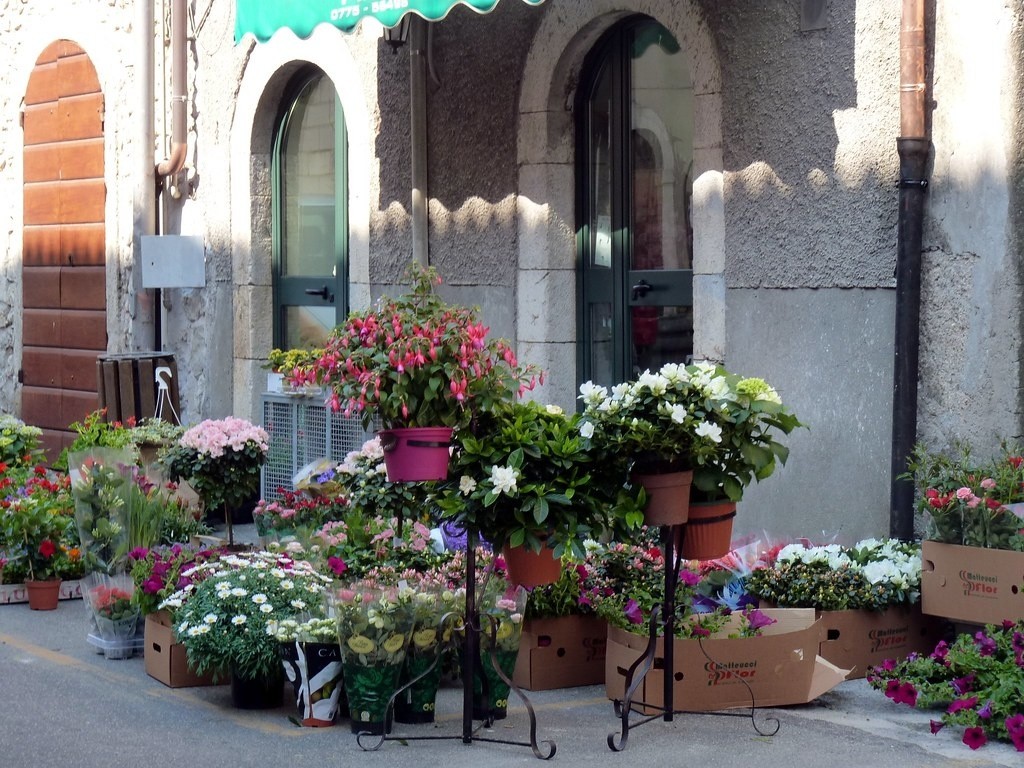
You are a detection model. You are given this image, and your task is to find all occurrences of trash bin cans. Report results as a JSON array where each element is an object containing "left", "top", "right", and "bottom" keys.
[{"left": 95, "top": 350, "right": 181, "bottom": 478}]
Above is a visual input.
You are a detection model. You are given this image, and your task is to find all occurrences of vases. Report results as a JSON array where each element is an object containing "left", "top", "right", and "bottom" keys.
[
  {"left": 499, "top": 525, "right": 562, "bottom": 587},
  {"left": 672, "top": 496, "right": 737, "bottom": 561},
  {"left": 633, "top": 466, "right": 695, "bottom": 527},
  {"left": 379, "top": 428, "right": 455, "bottom": 483},
  {"left": 25, "top": 577, "right": 62, "bottom": 611}
]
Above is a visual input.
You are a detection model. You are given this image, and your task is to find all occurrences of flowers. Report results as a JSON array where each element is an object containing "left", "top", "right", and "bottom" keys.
[{"left": 0, "top": 264, "right": 1024, "bottom": 754}]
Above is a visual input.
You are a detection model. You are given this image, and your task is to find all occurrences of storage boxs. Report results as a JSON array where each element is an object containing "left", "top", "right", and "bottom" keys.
[
  {"left": 602, "top": 607, "right": 856, "bottom": 712},
  {"left": 512, "top": 614, "right": 607, "bottom": 690},
  {"left": 144, "top": 612, "right": 231, "bottom": 688},
  {"left": 921, "top": 538, "right": 1024, "bottom": 628},
  {"left": 755, "top": 601, "right": 938, "bottom": 681}
]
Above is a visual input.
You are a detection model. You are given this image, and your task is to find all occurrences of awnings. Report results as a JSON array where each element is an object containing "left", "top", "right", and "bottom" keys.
[{"left": 234, "top": 0, "right": 548, "bottom": 45}]
